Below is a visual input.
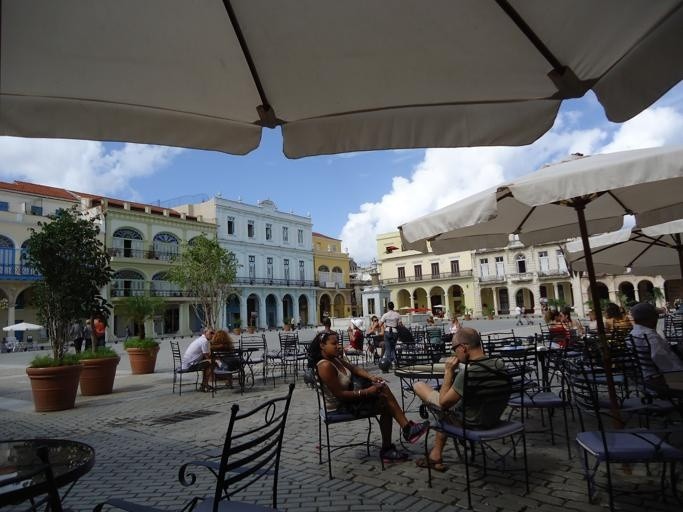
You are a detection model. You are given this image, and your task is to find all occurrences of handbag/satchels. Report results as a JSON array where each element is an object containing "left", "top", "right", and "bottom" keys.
[
  {"left": 221, "top": 355, "right": 241, "bottom": 371},
  {"left": 335, "top": 373, "right": 371, "bottom": 414}
]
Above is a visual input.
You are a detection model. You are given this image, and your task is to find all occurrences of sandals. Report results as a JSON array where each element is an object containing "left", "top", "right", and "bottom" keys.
[{"left": 416, "top": 456, "right": 445, "bottom": 471}]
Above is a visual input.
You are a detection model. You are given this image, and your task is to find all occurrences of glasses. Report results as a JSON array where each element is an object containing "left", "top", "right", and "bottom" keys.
[
  {"left": 450, "top": 342, "right": 468, "bottom": 352},
  {"left": 373, "top": 319, "right": 378, "bottom": 322}
]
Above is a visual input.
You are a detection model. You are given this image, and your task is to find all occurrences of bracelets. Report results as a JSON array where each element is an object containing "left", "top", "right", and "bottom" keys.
[{"left": 352, "top": 389, "right": 368, "bottom": 399}]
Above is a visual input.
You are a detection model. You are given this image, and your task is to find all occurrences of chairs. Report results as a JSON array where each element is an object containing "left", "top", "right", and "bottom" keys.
[
  {"left": 96, "top": 382, "right": 295, "bottom": 510},
  {"left": 170, "top": 318, "right": 681, "bottom": 511}
]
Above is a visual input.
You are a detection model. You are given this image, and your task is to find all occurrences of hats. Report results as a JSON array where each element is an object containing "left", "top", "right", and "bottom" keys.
[
  {"left": 632, "top": 301, "right": 664, "bottom": 320},
  {"left": 350, "top": 317, "right": 366, "bottom": 331}
]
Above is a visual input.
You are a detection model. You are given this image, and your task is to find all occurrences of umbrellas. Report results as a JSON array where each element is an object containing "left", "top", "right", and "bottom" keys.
[
  {"left": 0, "top": 1, "right": 683, "bottom": 165},
  {"left": 564, "top": 216, "right": 683, "bottom": 287},
  {"left": 2, "top": 321, "right": 45, "bottom": 342},
  {"left": 394, "top": 144, "right": 683, "bottom": 475}
]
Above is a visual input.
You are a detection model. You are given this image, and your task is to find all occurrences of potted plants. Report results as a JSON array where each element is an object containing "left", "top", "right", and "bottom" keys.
[
  {"left": 19, "top": 207, "right": 114, "bottom": 410},
  {"left": 169, "top": 236, "right": 235, "bottom": 347},
  {"left": 77, "top": 296, "right": 121, "bottom": 396},
  {"left": 115, "top": 288, "right": 169, "bottom": 374}
]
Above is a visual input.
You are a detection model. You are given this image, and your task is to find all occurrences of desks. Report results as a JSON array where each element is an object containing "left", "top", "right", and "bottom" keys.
[{"left": 0, "top": 439, "right": 95, "bottom": 511}]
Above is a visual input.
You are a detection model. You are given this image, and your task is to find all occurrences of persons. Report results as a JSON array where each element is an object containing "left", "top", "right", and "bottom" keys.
[
  {"left": 124, "top": 325, "right": 130, "bottom": 342},
  {"left": 410, "top": 326, "right": 517, "bottom": 474},
  {"left": 94, "top": 318, "right": 105, "bottom": 346},
  {"left": 515, "top": 302, "right": 683, "bottom": 399},
  {"left": 83, "top": 319, "right": 92, "bottom": 352},
  {"left": 320, "top": 316, "right": 339, "bottom": 337},
  {"left": 69, "top": 318, "right": 84, "bottom": 354},
  {"left": 342, "top": 300, "right": 464, "bottom": 372},
  {"left": 179, "top": 326, "right": 216, "bottom": 393},
  {"left": 210, "top": 327, "right": 246, "bottom": 388},
  {"left": 304, "top": 329, "right": 432, "bottom": 464}
]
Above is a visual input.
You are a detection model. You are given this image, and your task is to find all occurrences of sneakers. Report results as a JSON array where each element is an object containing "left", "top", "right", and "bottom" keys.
[
  {"left": 200, "top": 384, "right": 214, "bottom": 392},
  {"left": 403, "top": 419, "right": 430, "bottom": 443},
  {"left": 380, "top": 444, "right": 408, "bottom": 462}
]
